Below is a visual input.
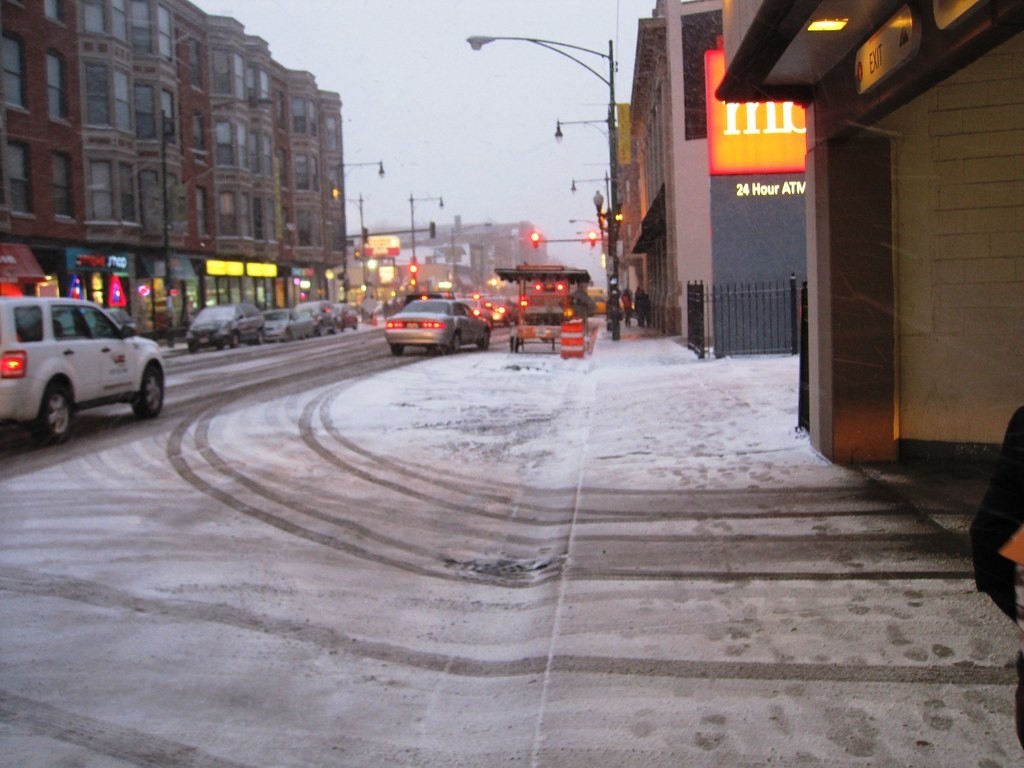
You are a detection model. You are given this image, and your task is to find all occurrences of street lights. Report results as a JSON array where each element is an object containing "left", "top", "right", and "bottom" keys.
[
  {"left": 318, "top": 160, "right": 386, "bottom": 299},
  {"left": 467, "top": 33, "right": 623, "bottom": 343},
  {"left": 407, "top": 193, "right": 445, "bottom": 297}
]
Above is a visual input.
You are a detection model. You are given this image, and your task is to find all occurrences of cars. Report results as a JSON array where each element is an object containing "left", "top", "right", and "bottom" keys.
[
  {"left": 186, "top": 302, "right": 266, "bottom": 354},
  {"left": 261, "top": 301, "right": 363, "bottom": 344},
  {"left": 470, "top": 294, "right": 519, "bottom": 329},
  {"left": 386, "top": 298, "right": 491, "bottom": 354}
]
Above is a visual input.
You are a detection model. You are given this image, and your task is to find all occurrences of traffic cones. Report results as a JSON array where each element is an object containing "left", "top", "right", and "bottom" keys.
[{"left": 373, "top": 310, "right": 381, "bottom": 327}]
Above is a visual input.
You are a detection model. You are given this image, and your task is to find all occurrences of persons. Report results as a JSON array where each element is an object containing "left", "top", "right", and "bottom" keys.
[{"left": 622, "top": 287, "right": 651, "bottom": 328}]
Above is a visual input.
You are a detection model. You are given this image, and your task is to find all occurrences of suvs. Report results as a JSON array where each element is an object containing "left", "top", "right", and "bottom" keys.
[{"left": 1, "top": 294, "right": 169, "bottom": 446}]
